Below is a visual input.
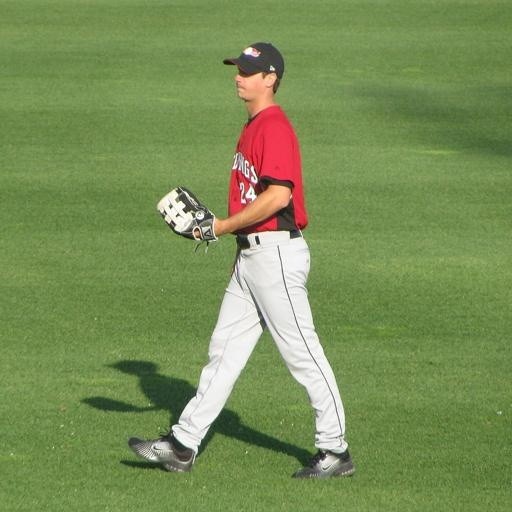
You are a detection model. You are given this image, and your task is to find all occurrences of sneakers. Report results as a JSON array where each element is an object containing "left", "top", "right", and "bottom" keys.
[
  {"left": 127, "top": 434, "right": 197, "bottom": 474},
  {"left": 290, "top": 451, "right": 356, "bottom": 480}
]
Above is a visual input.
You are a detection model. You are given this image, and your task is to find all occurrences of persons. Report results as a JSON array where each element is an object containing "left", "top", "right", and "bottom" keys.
[{"left": 126, "top": 42, "right": 356, "bottom": 479}]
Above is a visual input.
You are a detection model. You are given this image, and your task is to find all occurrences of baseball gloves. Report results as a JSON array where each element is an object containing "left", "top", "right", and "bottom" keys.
[{"left": 157, "top": 187, "right": 217, "bottom": 243}]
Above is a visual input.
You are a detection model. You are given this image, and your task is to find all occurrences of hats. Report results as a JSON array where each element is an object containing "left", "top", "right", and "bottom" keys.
[{"left": 222, "top": 41, "right": 285, "bottom": 76}]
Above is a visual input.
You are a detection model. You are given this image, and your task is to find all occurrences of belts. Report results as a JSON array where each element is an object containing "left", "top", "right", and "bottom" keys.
[{"left": 235, "top": 229, "right": 302, "bottom": 248}]
